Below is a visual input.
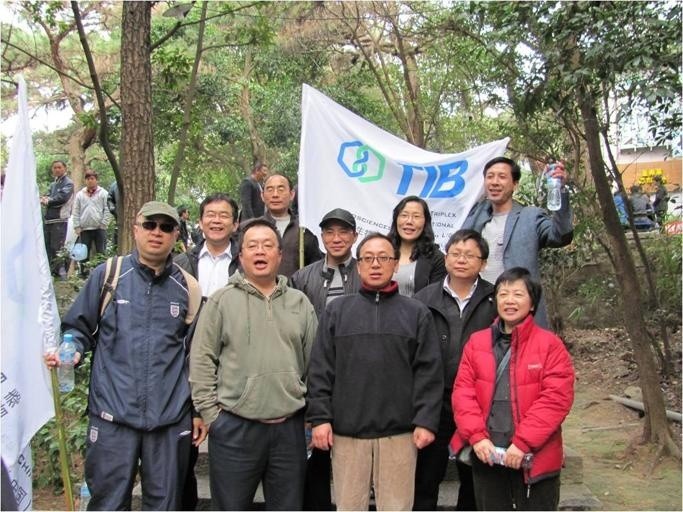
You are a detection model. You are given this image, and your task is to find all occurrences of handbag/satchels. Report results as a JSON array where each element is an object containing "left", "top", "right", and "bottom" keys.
[{"left": 69, "top": 234, "right": 87, "bottom": 261}]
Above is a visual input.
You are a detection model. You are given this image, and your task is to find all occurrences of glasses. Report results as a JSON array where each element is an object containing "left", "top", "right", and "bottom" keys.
[
  {"left": 136, "top": 222, "right": 179, "bottom": 233},
  {"left": 358, "top": 256, "right": 396, "bottom": 263},
  {"left": 447, "top": 252, "right": 483, "bottom": 260}
]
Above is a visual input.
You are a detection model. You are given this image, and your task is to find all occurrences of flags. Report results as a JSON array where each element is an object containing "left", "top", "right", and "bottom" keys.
[
  {"left": 1, "top": 73, "right": 61, "bottom": 512},
  {"left": 297, "top": 83, "right": 511, "bottom": 261}
]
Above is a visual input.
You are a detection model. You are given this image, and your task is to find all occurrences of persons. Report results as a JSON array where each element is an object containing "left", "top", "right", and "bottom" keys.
[{"left": 614, "top": 173, "right": 673, "bottom": 233}]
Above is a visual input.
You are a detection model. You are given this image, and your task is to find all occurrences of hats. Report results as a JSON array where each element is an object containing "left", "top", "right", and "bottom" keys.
[
  {"left": 138, "top": 201, "right": 181, "bottom": 221},
  {"left": 318, "top": 208, "right": 356, "bottom": 228}
]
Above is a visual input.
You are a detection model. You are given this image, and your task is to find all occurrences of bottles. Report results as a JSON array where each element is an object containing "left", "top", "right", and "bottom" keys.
[
  {"left": 545, "top": 165, "right": 561, "bottom": 211},
  {"left": 78, "top": 477, "right": 91, "bottom": 511},
  {"left": 302, "top": 419, "right": 313, "bottom": 461},
  {"left": 490, "top": 446, "right": 533, "bottom": 471},
  {"left": 55, "top": 332, "right": 75, "bottom": 392}
]
